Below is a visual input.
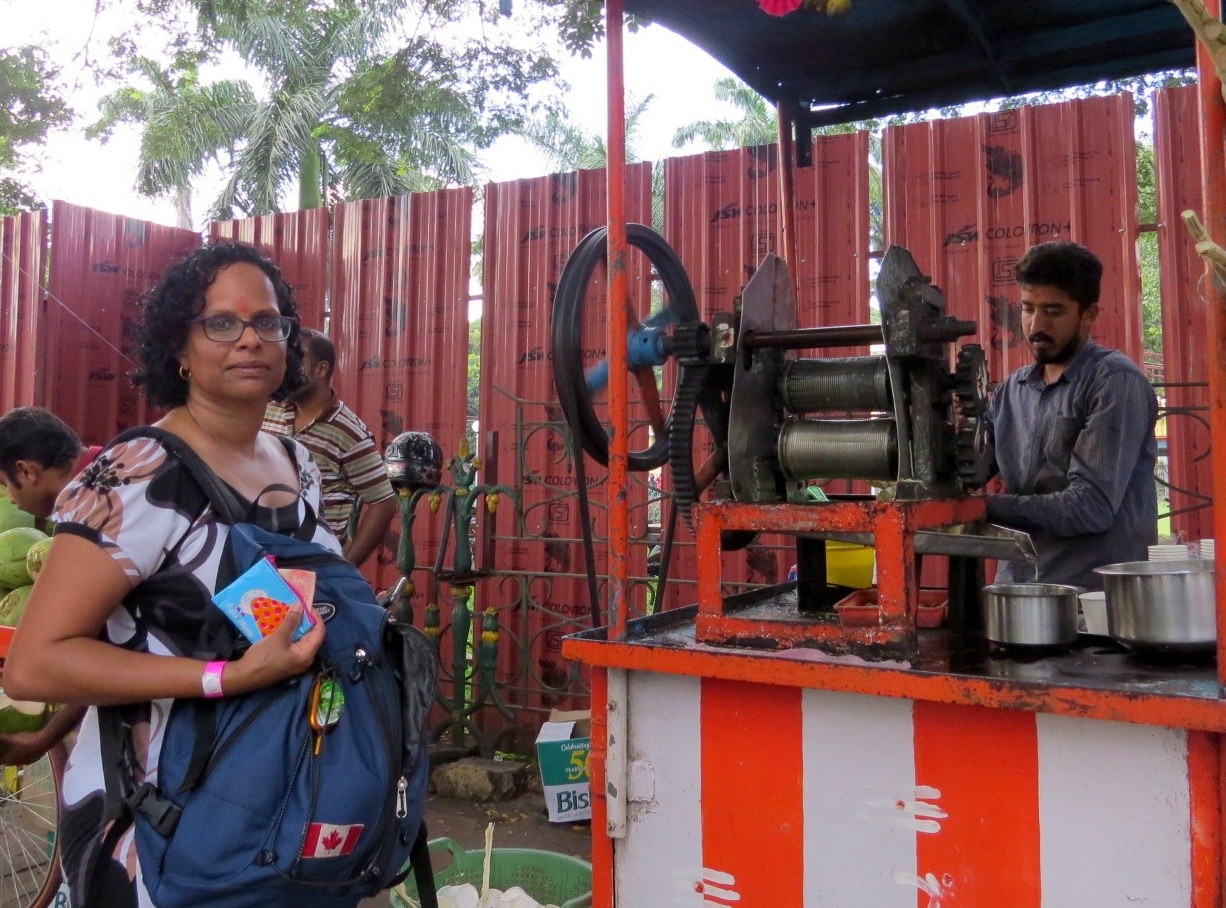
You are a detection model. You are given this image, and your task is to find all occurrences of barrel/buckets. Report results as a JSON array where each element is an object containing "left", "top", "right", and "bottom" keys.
[{"left": 825, "top": 540, "right": 875, "bottom": 588}]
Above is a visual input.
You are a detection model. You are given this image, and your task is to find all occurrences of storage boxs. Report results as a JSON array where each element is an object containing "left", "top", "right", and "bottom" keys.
[{"left": 537, "top": 708, "right": 592, "bottom": 823}]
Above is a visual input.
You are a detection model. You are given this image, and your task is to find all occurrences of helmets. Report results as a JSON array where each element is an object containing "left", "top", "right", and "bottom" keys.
[{"left": 383, "top": 432, "right": 442, "bottom": 484}]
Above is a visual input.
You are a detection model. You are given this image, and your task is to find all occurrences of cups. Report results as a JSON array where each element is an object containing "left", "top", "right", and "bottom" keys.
[
  {"left": 1147, "top": 538, "right": 1215, "bottom": 562},
  {"left": 1079, "top": 591, "right": 1106, "bottom": 634}
]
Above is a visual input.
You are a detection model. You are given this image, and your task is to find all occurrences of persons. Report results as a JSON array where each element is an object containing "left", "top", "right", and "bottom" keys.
[
  {"left": 973, "top": 242, "right": 1158, "bottom": 612},
  {"left": 0, "top": 242, "right": 397, "bottom": 908}
]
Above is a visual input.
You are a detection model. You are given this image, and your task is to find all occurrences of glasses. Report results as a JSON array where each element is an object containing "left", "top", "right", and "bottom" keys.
[{"left": 190, "top": 312, "right": 294, "bottom": 341}]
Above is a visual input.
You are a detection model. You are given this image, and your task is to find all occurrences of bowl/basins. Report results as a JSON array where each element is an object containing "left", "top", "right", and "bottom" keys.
[
  {"left": 982, "top": 584, "right": 1084, "bottom": 661},
  {"left": 1093, "top": 561, "right": 1216, "bottom": 668}
]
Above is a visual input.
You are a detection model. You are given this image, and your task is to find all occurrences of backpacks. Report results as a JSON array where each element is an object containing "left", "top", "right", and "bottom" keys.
[{"left": 96, "top": 425, "right": 438, "bottom": 908}]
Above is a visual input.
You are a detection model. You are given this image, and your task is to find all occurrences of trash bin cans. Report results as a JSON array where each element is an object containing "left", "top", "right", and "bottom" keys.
[{"left": 389, "top": 837, "right": 593, "bottom": 907}]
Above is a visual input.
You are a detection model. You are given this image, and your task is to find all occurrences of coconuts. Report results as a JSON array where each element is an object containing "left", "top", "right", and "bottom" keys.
[{"left": 0, "top": 482, "right": 69, "bottom": 733}]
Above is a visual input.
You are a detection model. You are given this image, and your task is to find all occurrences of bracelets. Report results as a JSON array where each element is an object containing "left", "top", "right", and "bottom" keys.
[{"left": 202, "top": 662, "right": 226, "bottom": 697}]
